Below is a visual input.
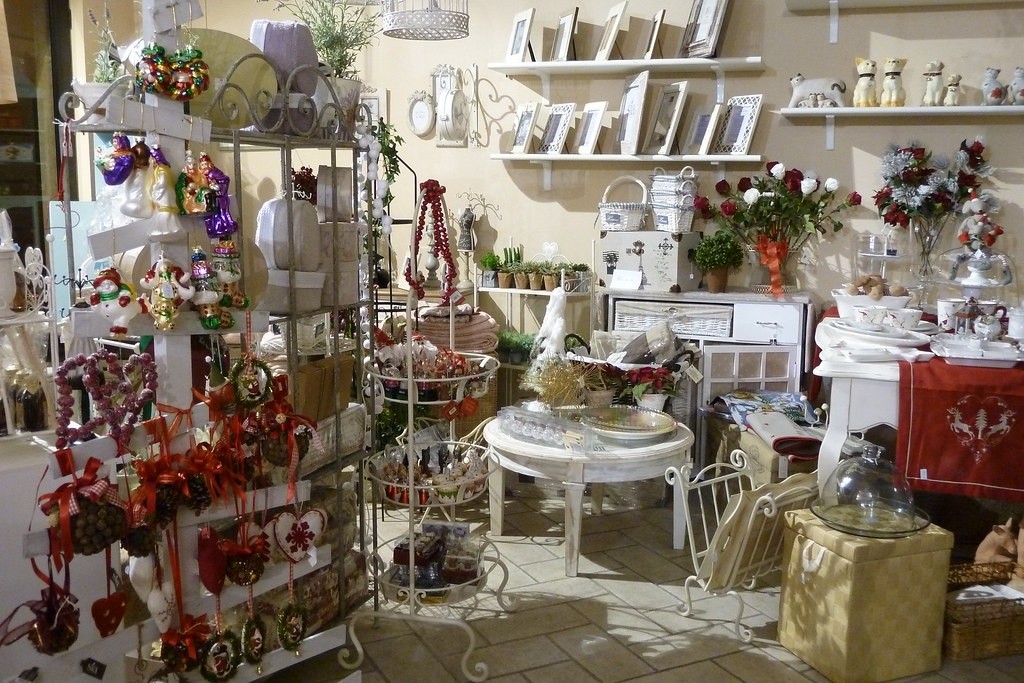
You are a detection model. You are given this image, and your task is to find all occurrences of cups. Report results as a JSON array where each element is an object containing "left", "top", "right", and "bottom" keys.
[
  {"left": 954, "top": 316, "right": 970, "bottom": 339},
  {"left": 966, "top": 300, "right": 1007, "bottom": 334},
  {"left": 937, "top": 298, "right": 967, "bottom": 333}
]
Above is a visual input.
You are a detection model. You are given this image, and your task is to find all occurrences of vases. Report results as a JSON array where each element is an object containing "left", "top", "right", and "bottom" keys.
[
  {"left": 747, "top": 246, "right": 798, "bottom": 285},
  {"left": 635, "top": 394, "right": 668, "bottom": 410}
]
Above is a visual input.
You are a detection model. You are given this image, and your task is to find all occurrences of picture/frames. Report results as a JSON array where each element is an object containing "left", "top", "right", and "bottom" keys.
[
  {"left": 509, "top": 69, "right": 762, "bottom": 157},
  {"left": 358, "top": 89, "right": 389, "bottom": 126},
  {"left": 504, "top": 0, "right": 729, "bottom": 62}
]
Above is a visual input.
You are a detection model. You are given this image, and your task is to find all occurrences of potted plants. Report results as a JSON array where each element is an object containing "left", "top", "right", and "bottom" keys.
[
  {"left": 690, "top": 231, "right": 743, "bottom": 291},
  {"left": 483, "top": 247, "right": 587, "bottom": 291},
  {"left": 496, "top": 331, "right": 579, "bottom": 364},
  {"left": 259, "top": 0, "right": 385, "bottom": 134},
  {"left": 73, "top": 57, "right": 128, "bottom": 122}
]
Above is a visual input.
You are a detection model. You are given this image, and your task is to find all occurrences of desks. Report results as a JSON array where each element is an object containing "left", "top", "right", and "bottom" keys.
[
  {"left": 483, "top": 407, "right": 695, "bottom": 578},
  {"left": 815, "top": 303, "right": 1024, "bottom": 511}
]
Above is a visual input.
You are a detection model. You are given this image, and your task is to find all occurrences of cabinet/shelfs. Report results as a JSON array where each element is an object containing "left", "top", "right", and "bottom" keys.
[
  {"left": 59, "top": 123, "right": 378, "bottom": 683},
  {"left": 606, "top": 290, "right": 813, "bottom": 476},
  {"left": 489, "top": 57, "right": 767, "bottom": 163}
]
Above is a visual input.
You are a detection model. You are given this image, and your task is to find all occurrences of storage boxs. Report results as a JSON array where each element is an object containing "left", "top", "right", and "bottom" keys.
[{"left": 592, "top": 231, "right": 702, "bottom": 292}]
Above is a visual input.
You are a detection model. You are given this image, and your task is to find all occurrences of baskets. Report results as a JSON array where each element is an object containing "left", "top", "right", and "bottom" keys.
[
  {"left": 648, "top": 166, "right": 699, "bottom": 234},
  {"left": 942, "top": 559, "right": 1024, "bottom": 661},
  {"left": 596, "top": 164, "right": 648, "bottom": 232}
]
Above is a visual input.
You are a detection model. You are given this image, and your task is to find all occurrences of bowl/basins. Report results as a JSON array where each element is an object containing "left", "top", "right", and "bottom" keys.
[
  {"left": 854, "top": 305, "right": 887, "bottom": 325},
  {"left": 887, "top": 308, "right": 923, "bottom": 328}
]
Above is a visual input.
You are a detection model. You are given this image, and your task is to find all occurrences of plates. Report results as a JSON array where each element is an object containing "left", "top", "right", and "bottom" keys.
[
  {"left": 882, "top": 317, "right": 933, "bottom": 330},
  {"left": 834, "top": 319, "right": 908, "bottom": 338},
  {"left": 820, "top": 321, "right": 932, "bottom": 348},
  {"left": 579, "top": 404, "right": 677, "bottom": 439},
  {"left": 914, "top": 323, "right": 945, "bottom": 336}
]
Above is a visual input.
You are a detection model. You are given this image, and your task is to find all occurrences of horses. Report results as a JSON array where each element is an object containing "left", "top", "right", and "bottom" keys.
[{"left": 975, "top": 517, "right": 1017, "bottom": 561}]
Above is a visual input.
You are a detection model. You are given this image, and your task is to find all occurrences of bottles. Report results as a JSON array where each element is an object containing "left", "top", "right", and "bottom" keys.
[{"left": 0, "top": 368, "right": 47, "bottom": 437}]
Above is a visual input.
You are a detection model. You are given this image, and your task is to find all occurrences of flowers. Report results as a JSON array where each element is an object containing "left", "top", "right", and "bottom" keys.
[
  {"left": 691, "top": 161, "right": 862, "bottom": 245},
  {"left": 626, "top": 370, "right": 679, "bottom": 397},
  {"left": 871, "top": 137, "right": 995, "bottom": 273}
]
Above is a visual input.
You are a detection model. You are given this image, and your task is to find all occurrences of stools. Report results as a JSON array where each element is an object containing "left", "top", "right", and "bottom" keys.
[{"left": 777, "top": 511, "right": 953, "bottom": 683}]
